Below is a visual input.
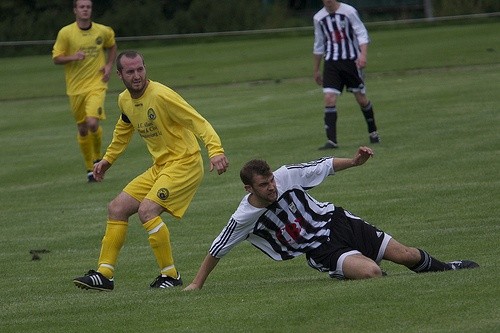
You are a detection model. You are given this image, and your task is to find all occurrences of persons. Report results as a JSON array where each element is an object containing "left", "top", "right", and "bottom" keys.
[
  {"left": 311, "top": 0, "right": 379, "bottom": 150},
  {"left": 72, "top": 51, "right": 229, "bottom": 291},
  {"left": 52, "top": 0, "right": 117, "bottom": 180},
  {"left": 182, "top": 146, "right": 480, "bottom": 291}
]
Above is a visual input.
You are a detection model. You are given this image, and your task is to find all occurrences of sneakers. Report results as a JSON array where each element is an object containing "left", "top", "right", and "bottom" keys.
[
  {"left": 87, "top": 170, "right": 96, "bottom": 182},
  {"left": 369, "top": 131, "right": 379, "bottom": 143},
  {"left": 73, "top": 270, "right": 114, "bottom": 291},
  {"left": 319, "top": 140, "right": 340, "bottom": 150},
  {"left": 150, "top": 272, "right": 183, "bottom": 289},
  {"left": 443, "top": 260, "right": 479, "bottom": 270}
]
